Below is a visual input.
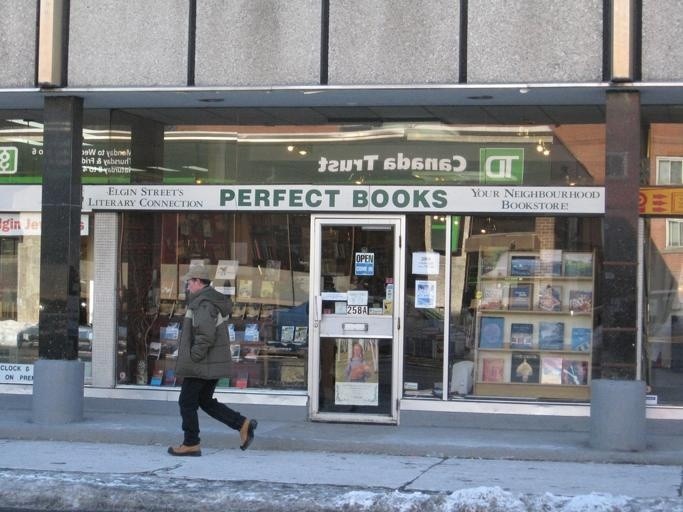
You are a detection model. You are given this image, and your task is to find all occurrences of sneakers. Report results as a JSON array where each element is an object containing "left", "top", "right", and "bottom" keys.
[
  {"left": 239, "top": 418, "right": 258, "bottom": 451},
  {"left": 168, "top": 443, "right": 202, "bottom": 457}
]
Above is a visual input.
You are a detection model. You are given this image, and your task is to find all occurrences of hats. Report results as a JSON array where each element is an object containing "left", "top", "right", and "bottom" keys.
[{"left": 183, "top": 266, "right": 210, "bottom": 281}]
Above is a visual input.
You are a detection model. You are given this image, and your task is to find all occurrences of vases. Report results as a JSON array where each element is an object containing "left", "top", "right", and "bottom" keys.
[{"left": 136, "top": 358, "right": 149, "bottom": 386}]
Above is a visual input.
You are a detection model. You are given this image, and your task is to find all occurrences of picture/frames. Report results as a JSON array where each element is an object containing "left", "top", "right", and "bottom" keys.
[{"left": 507, "top": 282, "right": 534, "bottom": 311}]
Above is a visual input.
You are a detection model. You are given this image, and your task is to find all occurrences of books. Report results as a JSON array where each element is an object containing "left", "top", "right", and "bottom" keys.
[
  {"left": 149, "top": 254, "right": 307, "bottom": 390},
  {"left": 479, "top": 246, "right": 593, "bottom": 385}
]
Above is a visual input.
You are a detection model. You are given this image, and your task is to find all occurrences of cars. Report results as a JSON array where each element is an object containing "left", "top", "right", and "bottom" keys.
[
  {"left": 272, "top": 293, "right": 473, "bottom": 382},
  {"left": 17, "top": 322, "right": 92, "bottom": 351}
]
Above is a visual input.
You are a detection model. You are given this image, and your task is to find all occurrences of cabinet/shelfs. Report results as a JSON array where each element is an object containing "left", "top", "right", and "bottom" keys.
[
  {"left": 473, "top": 246, "right": 596, "bottom": 400},
  {"left": 148, "top": 315, "right": 272, "bottom": 389}
]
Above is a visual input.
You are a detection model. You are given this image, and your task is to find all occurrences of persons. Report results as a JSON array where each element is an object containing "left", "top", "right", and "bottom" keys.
[
  {"left": 166, "top": 266, "right": 259, "bottom": 457},
  {"left": 343, "top": 342, "right": 371, "bottom": 382}
]
